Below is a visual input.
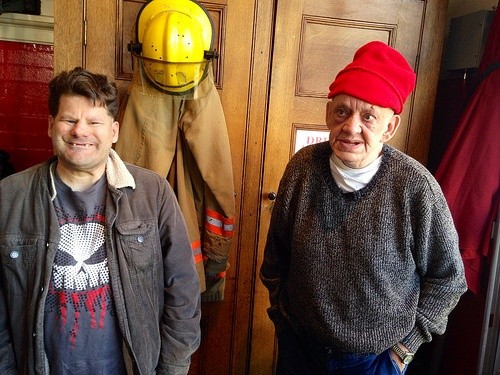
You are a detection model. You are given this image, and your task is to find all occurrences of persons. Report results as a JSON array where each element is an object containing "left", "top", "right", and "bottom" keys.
[
  {"left": 0, "top": 66, "right": 203, "bottom": 375},
  {"left": 260, "top": 41, "right": 469, "bottom": 375}
]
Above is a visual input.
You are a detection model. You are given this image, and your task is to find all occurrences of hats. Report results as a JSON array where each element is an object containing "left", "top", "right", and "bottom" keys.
[{"left": 326, "top": 40, "right": 418, "bottom": 114}]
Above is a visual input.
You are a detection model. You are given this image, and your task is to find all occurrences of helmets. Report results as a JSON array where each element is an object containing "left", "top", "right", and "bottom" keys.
[{"left": 128, "top": 0, "right": 219, "bottom": 99}]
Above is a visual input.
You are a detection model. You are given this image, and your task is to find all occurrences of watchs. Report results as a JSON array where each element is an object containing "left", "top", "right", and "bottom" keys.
[{"left": 393, "top": 345, "right": 414, "bottom": 365}]
